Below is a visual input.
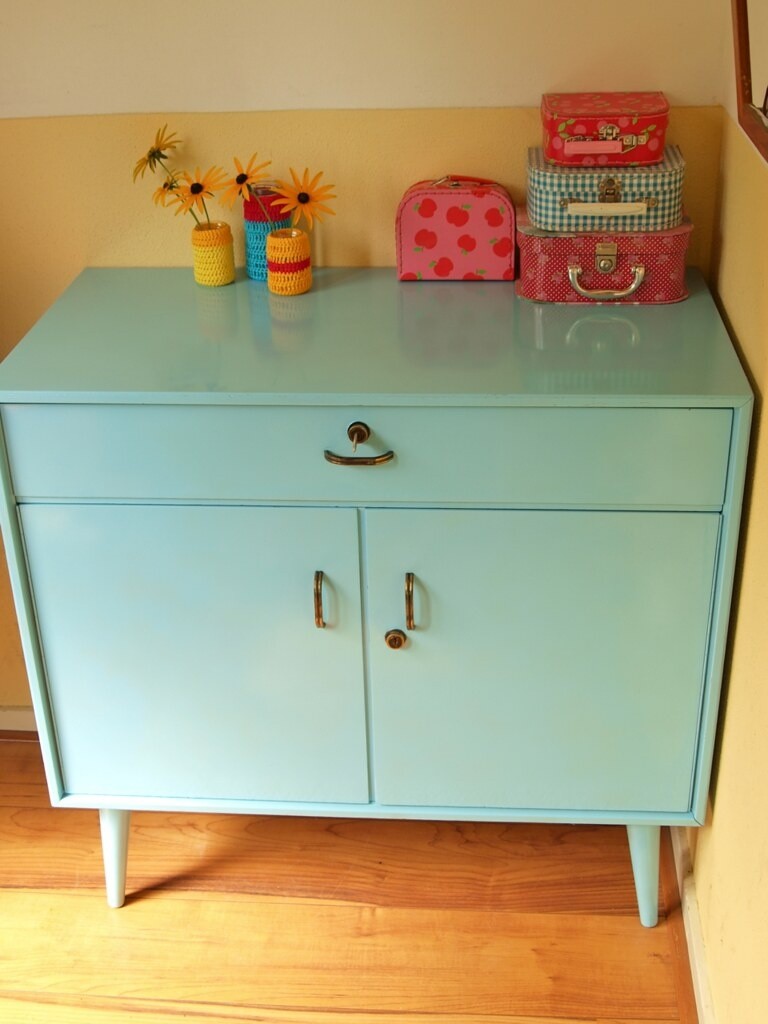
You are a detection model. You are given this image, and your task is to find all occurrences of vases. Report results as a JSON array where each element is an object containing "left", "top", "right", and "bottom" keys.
[
  {"left": 266, "top": 228, "right": 313, "bottom": 296},
  {"left": 242, "top": 184, "right": 293, "bottom": 283},
  {"left": 191, "top": 219, "right": 236, "bottom": 287}
]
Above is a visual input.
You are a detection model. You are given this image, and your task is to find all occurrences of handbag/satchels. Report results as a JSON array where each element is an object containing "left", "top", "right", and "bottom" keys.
[
  {"left": 394, "top": 174, "right": 515, "bottom": 280},
  {"left": 525, "top": 146, "right": 686, "bottom": 232},
  {"left": 539, "top": 91, "right": 670, "bottom": 167}
]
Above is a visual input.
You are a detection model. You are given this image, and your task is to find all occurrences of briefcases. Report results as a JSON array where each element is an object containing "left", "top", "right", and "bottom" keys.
[{"left": 513, "top": 202, "right": 694, "bottom": 304}]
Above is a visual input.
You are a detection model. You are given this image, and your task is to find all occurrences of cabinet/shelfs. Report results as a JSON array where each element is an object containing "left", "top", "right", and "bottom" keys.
[{"left": 0, "top": 266, "right": 758, "bottom": 929}]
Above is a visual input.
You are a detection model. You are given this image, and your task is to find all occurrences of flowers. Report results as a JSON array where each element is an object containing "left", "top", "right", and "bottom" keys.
[
  {"left": 130, "top": 121, "right": 232, "bottom": 229},
  {"left": 218, "top": 151, "right": 338, "bottom": 238}
]
